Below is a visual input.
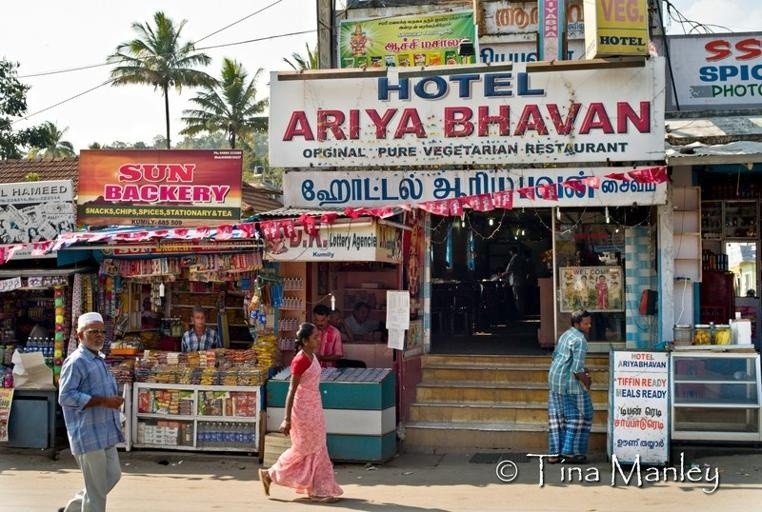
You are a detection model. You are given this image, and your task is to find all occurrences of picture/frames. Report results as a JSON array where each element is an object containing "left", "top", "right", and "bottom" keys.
[{"left": 557, "top": 265, "right": 626, "bottom": 314}]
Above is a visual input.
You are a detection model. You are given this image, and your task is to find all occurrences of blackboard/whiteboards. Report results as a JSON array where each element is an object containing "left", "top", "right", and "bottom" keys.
[{"left": 607, "top": 344, "right": 671, "bottom": 468}]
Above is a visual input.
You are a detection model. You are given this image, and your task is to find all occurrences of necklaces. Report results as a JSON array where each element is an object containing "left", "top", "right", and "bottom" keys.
[{"left": 302, "top": 351, "right": 311, "bottom": 363}]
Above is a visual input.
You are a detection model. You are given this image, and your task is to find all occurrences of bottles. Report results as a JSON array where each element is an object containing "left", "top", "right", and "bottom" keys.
[
  {"left": 712, "top": 325, "right": 731, "bottom": 352},
  {"left": 672, "top": 323, "right": 693, "bottom": 351},
  {"left": 701, "top": 253, "right": 727, "bottom": 271},
  {"left": 183, "top": 423, "right": 254, "bottom": 448},
  {"left": 0, "top": 320, "right": 14, "bottom": 389},
  {"left": 23, "top": 336, "right": 54, "bottom": 370},
  {"left": 693, "top": 324, "right": 711, "bottom": 351},
  {"left": 134, "top": 356, "right": 141, "bottom": 368}
]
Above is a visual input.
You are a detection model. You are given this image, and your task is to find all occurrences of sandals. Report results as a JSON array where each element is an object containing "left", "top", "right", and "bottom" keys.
[
  {"left": 548, "top": 454, "right": 587, "bottom": 463},
  {"left": 259, "top": 469, "right": 272, "bottom": 496},
  {"left": 311, "top": 496, "right": 338, "bottom": 503}
]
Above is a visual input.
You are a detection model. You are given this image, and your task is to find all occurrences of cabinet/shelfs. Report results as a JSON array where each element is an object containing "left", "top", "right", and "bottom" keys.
[
  {"left": 340, "top": 288, "right": 392, "bottom": 314},
  {"left": 668, "top": 183, "right": 757, "bottom": 323},
  {"left": 132, "top": 381, "right": 263, "bottom": 456},
  {"left": 669, "top": 352, "right": 762, "bottom": 447},
  {"left": 109, "top": 383, "right": 132, "bottom": 453},
  {"left": 275, "top": 287, "right": 304, "bottom": 353},
  {"left": 164, "top": 278, "right": 254, "bottom": 350}
]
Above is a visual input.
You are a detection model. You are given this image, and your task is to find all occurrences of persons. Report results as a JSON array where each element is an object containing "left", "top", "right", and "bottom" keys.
[
  {"left": 501, "top": 246, "right": 524, "bottom": 319},
  {"left": 328, "top": 306, "right": 353, "bottom": 343},
  {"left": 259, "top": 322, "right": 343, "bottom": 503},
  {"left": 595, "top": 275, "right": 608, "bottom": 309},
  {"left": 548, "top": 310, "right": 594, "bottom": 464},
  {"left": 16, "top": 315, "right": 49, "bottom": 338},
  {"left": 577, "top": 275, "right": 589, "bottom": 306},
  {"left": 345, "top": 302, "right": 374, "bottom": 341},
  {"left": 180, "top": 307, "right": 222, "bottom": 351},
  {"left": 311, "top": 305, "right": 344, "bottom": 368},
  {"left": 58, "top": 312, "right": 125, "bottom": 512}
]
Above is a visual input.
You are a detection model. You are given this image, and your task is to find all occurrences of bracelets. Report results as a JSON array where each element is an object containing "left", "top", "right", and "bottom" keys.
[{"left": 284, "top": 416, "right": 291, "bottom": 422}]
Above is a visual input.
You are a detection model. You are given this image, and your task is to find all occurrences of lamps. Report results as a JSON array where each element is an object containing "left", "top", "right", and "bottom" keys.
[
  {"left": 456, "top": 37, "right": 475, "bottom": 65},
  {"left": 306, "top": 292, "right": 336, "bottom": 311}
]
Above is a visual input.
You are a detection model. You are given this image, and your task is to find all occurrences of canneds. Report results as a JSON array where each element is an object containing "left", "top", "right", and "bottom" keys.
[{"left": 672, "top": 325, "right": 732, "bottom": 347}]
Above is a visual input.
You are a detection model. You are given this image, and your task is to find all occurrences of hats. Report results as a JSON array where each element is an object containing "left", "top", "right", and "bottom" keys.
[{"left": 78, "top": 312, "right": 104, "bottom": 329}]
